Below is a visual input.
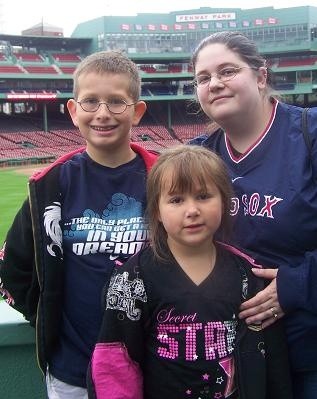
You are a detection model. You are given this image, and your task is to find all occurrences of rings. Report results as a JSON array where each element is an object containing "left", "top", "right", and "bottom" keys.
[{"left": 271, "top": 307, "right": 277, "bottom": 317}]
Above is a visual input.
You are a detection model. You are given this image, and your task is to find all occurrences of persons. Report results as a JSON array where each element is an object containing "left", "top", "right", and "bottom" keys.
[
  {"left": 86, "top": 144, "right": 292, "bottom": 399},
  {"left": 1, "top": 51, "right": 162, "bottom": 399},
  {"left": 188, "top": 32, "right": 317, "bottom": 399}
]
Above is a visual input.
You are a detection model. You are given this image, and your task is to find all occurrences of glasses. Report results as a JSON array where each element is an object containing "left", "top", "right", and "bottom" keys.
[
  {"left": 74, "top": 98, "right": 136, "bottom": 114},
  {"left": 190, "top": 65, "right": 260, "bottom": 89}
]
